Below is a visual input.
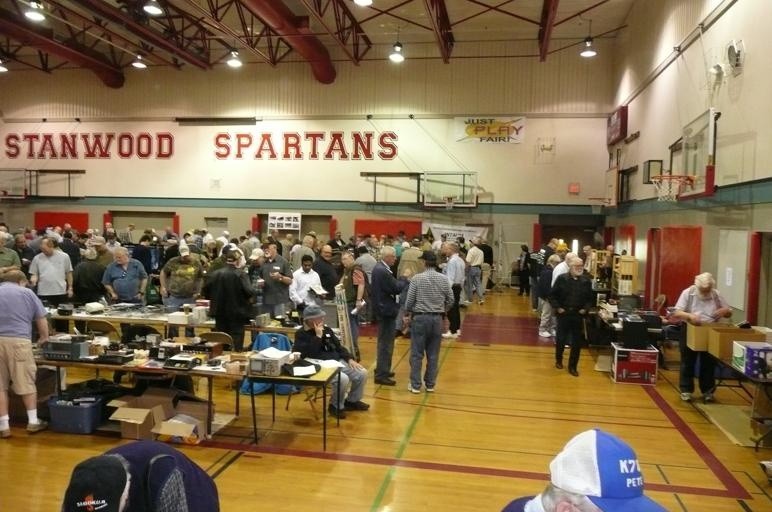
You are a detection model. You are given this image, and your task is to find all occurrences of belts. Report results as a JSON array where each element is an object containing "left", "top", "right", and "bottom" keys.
[{"left": 415, "top": 311, "right": 445, "bottom": 316}]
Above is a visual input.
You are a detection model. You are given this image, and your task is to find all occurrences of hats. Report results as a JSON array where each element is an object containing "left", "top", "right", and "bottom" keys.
[
  {"left": 557, "top": 243, "right": 570, "bottom": 252},
  {"left": 262, "top": 236, "right": 276, "bottom": 244},
  {"left": 226, "top": 250, "right": 243, "bottom": 262},
  {"left": 418, "top": 253, "right": 436, "bottom": 261},
  {"left": 65, "top": 455, "right": 127, "bottom": 511},
  {"left": 179, "top": 244, "right": 190, "bottom": 256},
  {"left": 92, "top": 236, "right": 104, "bottom": 245},
  {"left": 4, "top": 270, "right": 31, "bottom": 287},
  {"left": 469, "top": 239, "right": 479, "bottom": 246},
  {"left": 249, "top": 248, "right": 264, "bottom": 260},
  {"left": 307, "top": 231, "right": 316, "bottom": 237},
  {"left": 549, "top": 429, "right": 668, "bottom": 512},
  {"left": 303, "top": 305, "right": 326, "bottom": 320}
]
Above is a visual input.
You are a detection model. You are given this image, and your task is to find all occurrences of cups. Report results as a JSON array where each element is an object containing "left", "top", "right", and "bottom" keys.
[
  {"left": 272, "top": 266, "right": 281, "bottom": 277},
  {"left": 184, "top": 304, "right": 190, "bottom": 315}
]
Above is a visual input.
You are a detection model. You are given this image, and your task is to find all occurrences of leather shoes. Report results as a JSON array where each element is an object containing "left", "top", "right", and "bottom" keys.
[
  {"left": 374, "top": 369, "right": 394, "bottom": 377},
  {"left": 375, "top": 377, "right": 396, "bottom": 385}
]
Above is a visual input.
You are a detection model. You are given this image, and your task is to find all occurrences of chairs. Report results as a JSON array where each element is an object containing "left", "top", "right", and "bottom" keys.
[
  {"left": 251, "top": 332, "right": 293, "bottom": 395},
  {"left": 649, "top": 294, "right": 665, "bottom": 313},
  {"left": 195, "top": 331, "right": 234, "bottom": 392},
  {"left": 49, "top": 395, "right": 102, "bottom": 436}
]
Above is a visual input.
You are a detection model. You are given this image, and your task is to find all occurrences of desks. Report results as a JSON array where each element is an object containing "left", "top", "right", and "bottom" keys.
[
  {"left": 246, "top": 358, "right": 342, "bottom": 453},
  {"left": 696, "top": 352, "right": 771, "bottom": 452},
  {"left": 599, "top": 309, "right": 661, "bottom": 350},
  {"left": 33, "top": 307, "right": 343, "bottom": 352},
  {"left": 32, "top": 341, "right": 255, "bottom": 442}
]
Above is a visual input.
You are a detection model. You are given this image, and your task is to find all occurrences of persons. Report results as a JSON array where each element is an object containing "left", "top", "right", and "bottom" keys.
[
  {"left": 1, "top": 222, "right": 493, "bottom": 361},
  {"left": 661, "top": 312, "right": 685, "bottom": 354},
  {"left": 1, "top": 268, "right": 50, "bottom": 437},
  {"left": 502, "top": 428, "right": 668, "bottom": 512},
  {"left": 370, "top": 245, "right": 410, "bottom": 385},
  {"left": 760, "top": 460, "right": 772, "bottom": 483},
  {"left": 517, "top": 238, "right": 617, "bottom": 377},
  {"left": 291, "top": 304, "right": 371, "bottom": 419},
  {"left": 63, "top": 440, "right": 219, "bottom": 511},
  {"left": 403, "top": 250, "right": 455, "bottom": 394},
  {"left": 674, "top": 272, "right": 732, "bottom": 402}
]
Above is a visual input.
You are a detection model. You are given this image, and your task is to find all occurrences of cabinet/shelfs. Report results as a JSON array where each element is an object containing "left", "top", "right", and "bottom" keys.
[
  {"left": 588, "top": 249, "right": 611, "bottom": 282},
  {"left": 611, "top": 256, "right": 638, "bottom": 296}
]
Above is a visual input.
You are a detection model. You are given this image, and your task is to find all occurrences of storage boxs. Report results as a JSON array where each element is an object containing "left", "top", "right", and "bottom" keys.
[
  {"left": 151, "top": 401, "right": 207, "bottom": 445},
  {"left": 610, "top": 342, "right": 659, "bottom": 386},
  {"left": 249, "top": 349, "right": 290, "bottom": 377},
  {"left": 686, "top": 321, "right": 771, "bottom": 379},
  {"left": 106, "top": 387, "right": 177, "bottom": 440}
]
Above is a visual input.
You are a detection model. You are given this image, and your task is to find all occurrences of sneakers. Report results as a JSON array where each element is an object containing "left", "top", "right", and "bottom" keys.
[
  {"left": 464, "top": 300, "right": 473, "bottom": 303},
  {"left": 556, "top": 362, "right": 563, "bottom": 369},
  {"left": 704, "top": 391, "right": 713, "bottom": 402},
  {"left": 424, "top": 382, "right": 434, "bottom": 391},
  {"left": 569, "top": 368, "right": 578, "bottom": 376},
  {"left": 328, "top": 404, "right": 345, "bottom": 419},
  {"left": 1, "top": 429, "right": 12, "bottom": 437},
  {"left": 442, "top": 329, "right": 461, "bottom": 339},
  {"left": 539, "top": 330, "right": 551, "bottom": 337},
  {"left": 680, "top": 391, "right": 691, "bottom": 401},
  {"left": 27, "top": 420, "right": 48, "bottom": 432},
  {"left": 408, "top": 383, "right": 420, "bottom": 393},
  {"left": 478, "top": 299, "right": 484, "bottom": 305},
  {"left": 345, "top": 398, "right": 369, "bottom": 410}
]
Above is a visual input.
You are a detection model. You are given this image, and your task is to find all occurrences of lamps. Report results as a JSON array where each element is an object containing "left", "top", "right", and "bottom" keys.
[
  {"left": 387, "top": 25, "right": 405, "bottom": 64},
  {"left": 143, "top": 1, "right": 163, "bottom": 18},
  {"left": 132, "top": 51, "right": 147, "bottom": 70},
  {"left": 578, "top": 12, "right": 597, "bottom": 59},
  {"left": 226, "top": 39, "right": 242, "bottom": 70}
]
